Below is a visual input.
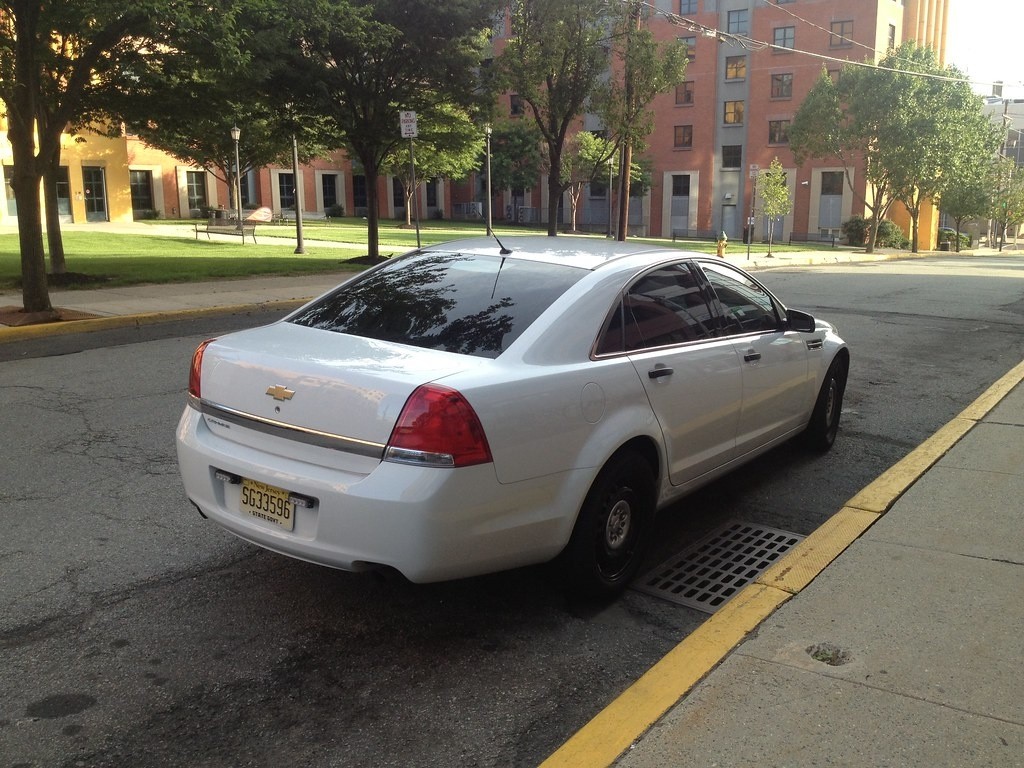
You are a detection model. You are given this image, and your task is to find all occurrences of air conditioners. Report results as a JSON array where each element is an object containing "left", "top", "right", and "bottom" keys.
[
  {"left": 519, "top": 206, "right": 530, "bottom": 223},
  {"left": 470, "top": 202, "right": 483, "bottom": 220},
  {"left": 505, "top": 204, "right": 519, "bottom": 222}
]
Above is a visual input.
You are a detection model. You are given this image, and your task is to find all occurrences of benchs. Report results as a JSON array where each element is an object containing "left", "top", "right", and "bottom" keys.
[
  {"left": 192, "top": 217, "right": 257, "bottom": 245},
  {"left": 672, "top": 228, "right": 717, "bottom": 243},
  {"left": 281, "top": 210, "right": 331, "bottom": 226},
  {"left": 233, "top": 210, "right": 280, "bottom": 226},
  {"left": 788, "top": 231, "right": 835, "bottom": 248}
]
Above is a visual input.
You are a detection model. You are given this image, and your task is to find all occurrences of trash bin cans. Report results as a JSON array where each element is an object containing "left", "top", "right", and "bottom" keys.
[
  {"left": 743, "top": 224, "right": 754, "bottom": 243},
  {"left": 214, "top": 209, "right": 230, "bottom": 226},
  {"left": 940, "top": 241, "right": 950, "bottom": 251}
]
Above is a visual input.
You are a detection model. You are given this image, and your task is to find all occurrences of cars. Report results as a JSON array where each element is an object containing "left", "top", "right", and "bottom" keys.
[
  {"left": 174, "top": 235, "right": 850, "bottom": 608},
  {"left": 941, "top": 227, "right": 967, "bottom": 236}
]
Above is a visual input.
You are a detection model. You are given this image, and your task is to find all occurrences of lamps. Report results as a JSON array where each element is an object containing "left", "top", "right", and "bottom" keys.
[{"left": 802, "top": 181, "right": 809, "bottom": 185}]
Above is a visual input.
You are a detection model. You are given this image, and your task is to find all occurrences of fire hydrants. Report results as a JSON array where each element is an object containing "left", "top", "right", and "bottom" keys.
[{"left": 717, "top": 231, "right": 728, "bottom": 258}]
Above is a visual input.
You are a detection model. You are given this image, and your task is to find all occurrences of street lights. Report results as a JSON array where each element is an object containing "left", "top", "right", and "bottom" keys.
[
  {"left": 286, "top": 102, "right": 308, "bottom": 255},
  {"left": 231, "top": 123, "right": 244, "bottom": 231},
  {"left": 607, "top": 156, "right": 613, "bottom": 238},
  {"left": 993, "top": 99, "right": 1024, "bottom": 249},
  {"left": 483, "top": 122, "right": 493, "bottom": 236}
]
